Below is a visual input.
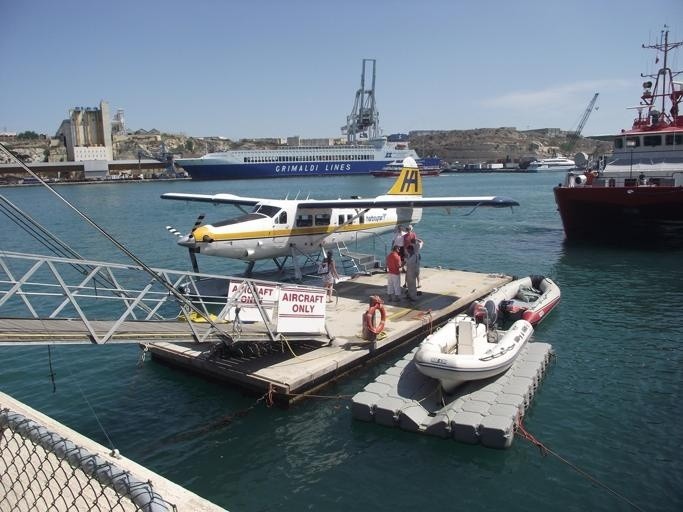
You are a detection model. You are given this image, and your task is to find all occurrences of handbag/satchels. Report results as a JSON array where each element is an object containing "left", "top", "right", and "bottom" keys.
[{"left": 317, "top": 262, "right": 329, "bottom": 275}]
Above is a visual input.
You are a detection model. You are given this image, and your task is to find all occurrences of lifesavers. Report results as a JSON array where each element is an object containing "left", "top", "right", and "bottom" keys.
[{"left": 365, "top": 304, "right": 386, "bottom": 334}]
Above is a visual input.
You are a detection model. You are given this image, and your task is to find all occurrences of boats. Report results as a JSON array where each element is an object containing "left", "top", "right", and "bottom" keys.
[
  {"left": 371, "top": 157, "right": 441, "bottom": 177},
  {"left": 417, "top": 273, "right": 561, "bottom": 395}
]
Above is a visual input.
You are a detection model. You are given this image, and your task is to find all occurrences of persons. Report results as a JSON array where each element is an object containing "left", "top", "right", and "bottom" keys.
[
  {"left": 322, "top": 249, "right": 339, "bottom": 302},
  {"left": 385, "top": 224, "right": 424, "bottom": 304},
  {"left": 639, "top": 172, "right": 646, "bottom": 180}
]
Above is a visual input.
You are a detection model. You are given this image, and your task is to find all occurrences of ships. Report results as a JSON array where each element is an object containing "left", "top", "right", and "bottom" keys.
[
  {"left": 553, "top": 23, "right": 682, "bottom": 250},
  {"left": 174, "top": 58, "right": 416, "bottom": 178}
]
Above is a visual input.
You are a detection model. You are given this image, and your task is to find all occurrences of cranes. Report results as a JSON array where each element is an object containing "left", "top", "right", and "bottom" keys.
[{"left": 561, "top": 93, "right": 599, "bottom": 158}]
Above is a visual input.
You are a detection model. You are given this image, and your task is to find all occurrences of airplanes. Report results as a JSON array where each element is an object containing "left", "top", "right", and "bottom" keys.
[{"left": 159, "top": 155, "right": 521, "bottom": 282}]
